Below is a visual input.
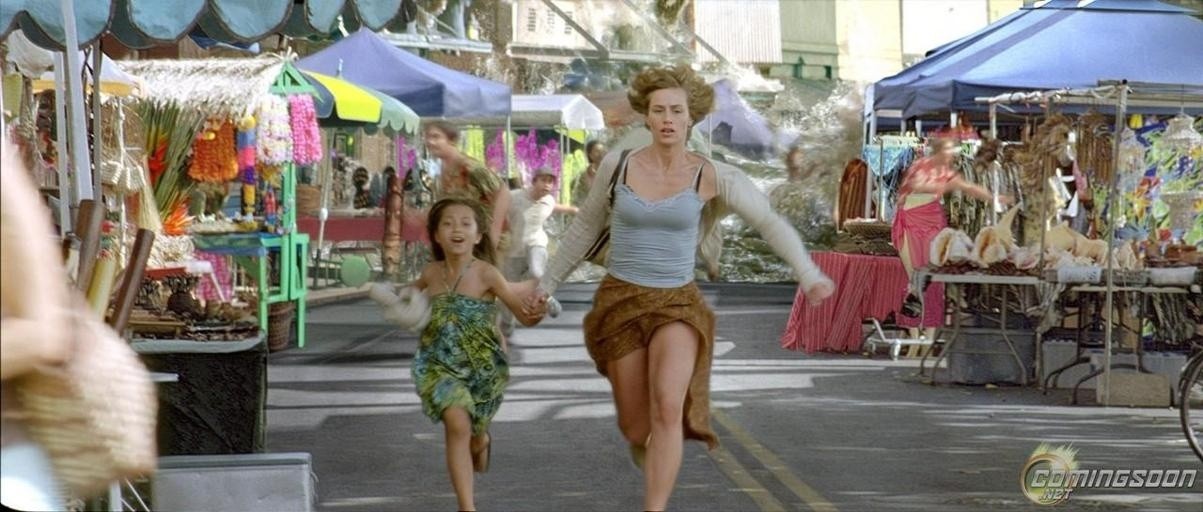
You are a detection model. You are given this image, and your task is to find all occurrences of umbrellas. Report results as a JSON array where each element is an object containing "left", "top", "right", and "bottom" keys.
[
  {"left": 312, "top": 56, "right": 423, "bottom": 286},
  {"left": 297, "top": 65, "right": 384, "bottom": 127}
]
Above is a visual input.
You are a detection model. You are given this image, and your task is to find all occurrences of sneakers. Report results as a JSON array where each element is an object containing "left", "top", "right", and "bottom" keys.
[{"left": 548, "top": 299, "right": 563, "bottom": 318}]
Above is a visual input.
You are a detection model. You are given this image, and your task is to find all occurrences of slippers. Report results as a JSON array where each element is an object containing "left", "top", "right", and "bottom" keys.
[{"left": 471, "top": 432, "right": 492, "bottom": 473}]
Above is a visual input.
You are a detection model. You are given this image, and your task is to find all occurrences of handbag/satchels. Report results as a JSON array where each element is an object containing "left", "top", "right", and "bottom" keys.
[
  {"left": 11, "top": 293, "right": 159, "bottom": 499},
  {"left": 585, "top": 224, "right": 610, "bottom": 266}
]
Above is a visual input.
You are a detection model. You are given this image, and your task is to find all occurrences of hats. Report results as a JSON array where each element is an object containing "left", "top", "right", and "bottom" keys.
[{"left": 532, "top": 167, "right": 558, "bottom": 187}]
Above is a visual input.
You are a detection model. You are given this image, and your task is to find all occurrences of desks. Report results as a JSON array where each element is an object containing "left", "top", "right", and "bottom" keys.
[
  {"left": 918, "top": 275, "right": 1040, "bottom": 388},
  {"left": 781, "top": 251, "right": 908, "bottom": 353},
  {"left": 297, "top": 215, "right": 431, "bottom": 286},
  {"left": 1046, "top": 285, "right": 1201, "bottom": 406}
]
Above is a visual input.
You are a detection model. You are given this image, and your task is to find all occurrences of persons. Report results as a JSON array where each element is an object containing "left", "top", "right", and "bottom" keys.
[
  {"left": 406, "top": 60, "right": 1022, "bottom": 512},
  {"left": 1, "top": 120, "right": 71, "bottom": 511}
]
